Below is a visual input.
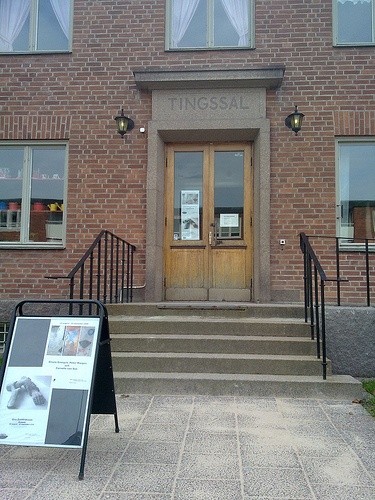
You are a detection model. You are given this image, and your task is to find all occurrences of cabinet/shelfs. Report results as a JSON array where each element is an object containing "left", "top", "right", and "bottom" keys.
[{"left": 0, "top": 166, "right": 65, "bottom": 243}]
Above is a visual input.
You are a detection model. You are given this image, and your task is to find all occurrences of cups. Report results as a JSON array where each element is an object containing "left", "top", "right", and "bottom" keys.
[
  {"left": 53, "top": 173, "right": 59, "bottom": 178},
  {"left": 48, "top": 204, "right": 57, "bottom": 210},
  {"left": 60, "top": 204, "right": 64, "bottom": 211},
  {"left": 42, "top": 173, "right": 49, "bottom": 178},
  {"left": 0, "top": 166, "right": 10, "bottom": 177}
]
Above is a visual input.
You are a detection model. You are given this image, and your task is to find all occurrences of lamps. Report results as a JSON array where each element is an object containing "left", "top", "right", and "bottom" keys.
[
  {"left": 285, "top": 104, "right": 305, "bottom": 136},
  {"left": 114, "top": 108, "right": 135, "bottom": 139}
]
